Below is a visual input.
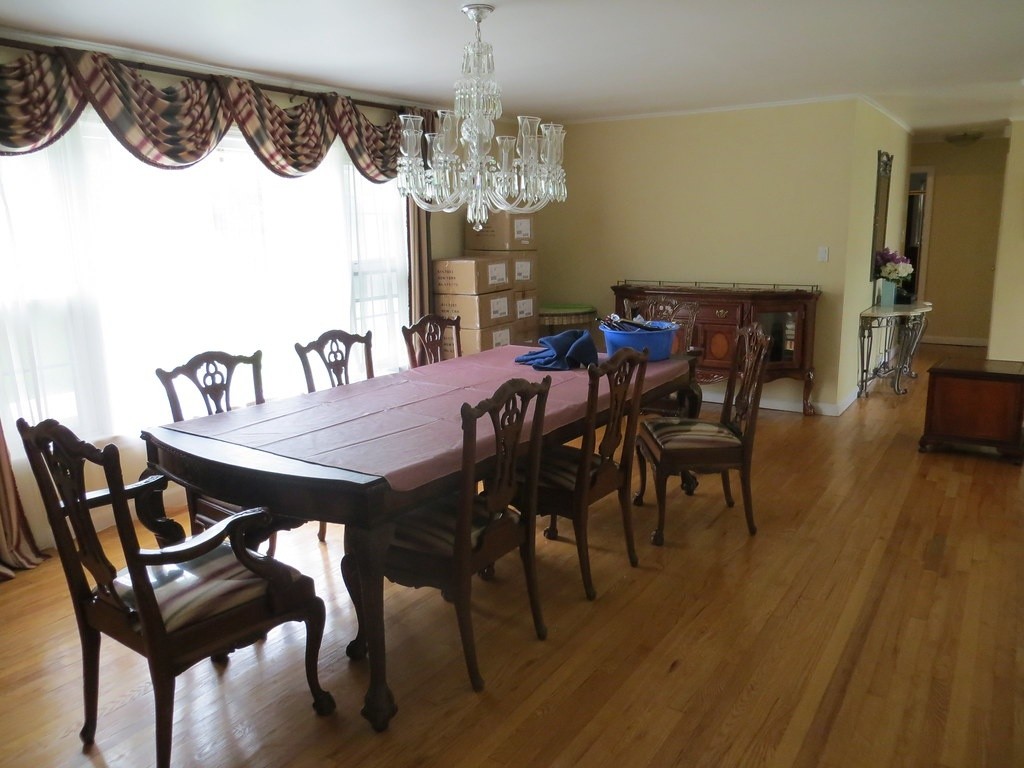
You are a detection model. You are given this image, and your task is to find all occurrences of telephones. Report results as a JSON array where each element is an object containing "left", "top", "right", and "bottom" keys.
[{"left": 897, "top": 286, "right": 916, "bottom": 304}]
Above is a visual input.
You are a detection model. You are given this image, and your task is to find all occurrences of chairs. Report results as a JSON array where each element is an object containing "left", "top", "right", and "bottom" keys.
[
  {"left": 298, "top": 328, "right": 374, "bottom": 392},
  {"left": 628, "top": 322, "right": 773, "bottom": 547},
  {"left": 472, "top": 348, "right": 650, "bottom": 602},
  {"left": 152, "top": 348, "right": 327, "bottom": 561},
  {"left": 16, "top": 416, "right": 336, "bottom": 768},
  {"left": 401, "top": 314, "right": 463, "bottom": 369},
  {"left": 343, "top": 373, "right": 549, "bottom": 693}
]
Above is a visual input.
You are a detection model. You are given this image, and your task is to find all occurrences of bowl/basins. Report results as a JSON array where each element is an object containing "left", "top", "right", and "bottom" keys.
[{"left": 598, "top": 321, "right": 681, "bottom": 361}]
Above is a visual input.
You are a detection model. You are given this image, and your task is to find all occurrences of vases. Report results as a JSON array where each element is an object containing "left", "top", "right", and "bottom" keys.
[{"left": 880, "top": 278, "right": 897, "bottom": 314}]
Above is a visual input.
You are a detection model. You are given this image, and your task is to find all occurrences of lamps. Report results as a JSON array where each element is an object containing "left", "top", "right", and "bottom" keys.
[{"left": 394, "top": 4, "right": 569, "bottom": 234}]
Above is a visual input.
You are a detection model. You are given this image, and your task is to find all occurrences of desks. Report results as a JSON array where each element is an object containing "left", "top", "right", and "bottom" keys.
[
  {"left": 857, "top": 300, "right": 932, "bottom": 399},
  {"left": 919, "top": 355, "right": 1023, "bottom": 466},
  {"left": 137, "top": 344, "right": 693, "bottom": 732}
]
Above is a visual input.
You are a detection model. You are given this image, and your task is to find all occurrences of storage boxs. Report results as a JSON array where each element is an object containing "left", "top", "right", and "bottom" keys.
[{"left": 430, "top": 207, "right": 537, "bottom": 362}]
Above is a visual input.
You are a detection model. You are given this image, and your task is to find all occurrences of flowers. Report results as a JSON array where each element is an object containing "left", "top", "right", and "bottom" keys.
[{"left": 873, "top": 247, "right": 913, "bottom": 285}]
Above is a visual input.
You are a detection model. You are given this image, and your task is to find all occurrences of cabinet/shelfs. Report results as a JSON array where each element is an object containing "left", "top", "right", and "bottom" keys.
[{"left": 611, "top": 283, "right": 821, "bottom": 418}]
